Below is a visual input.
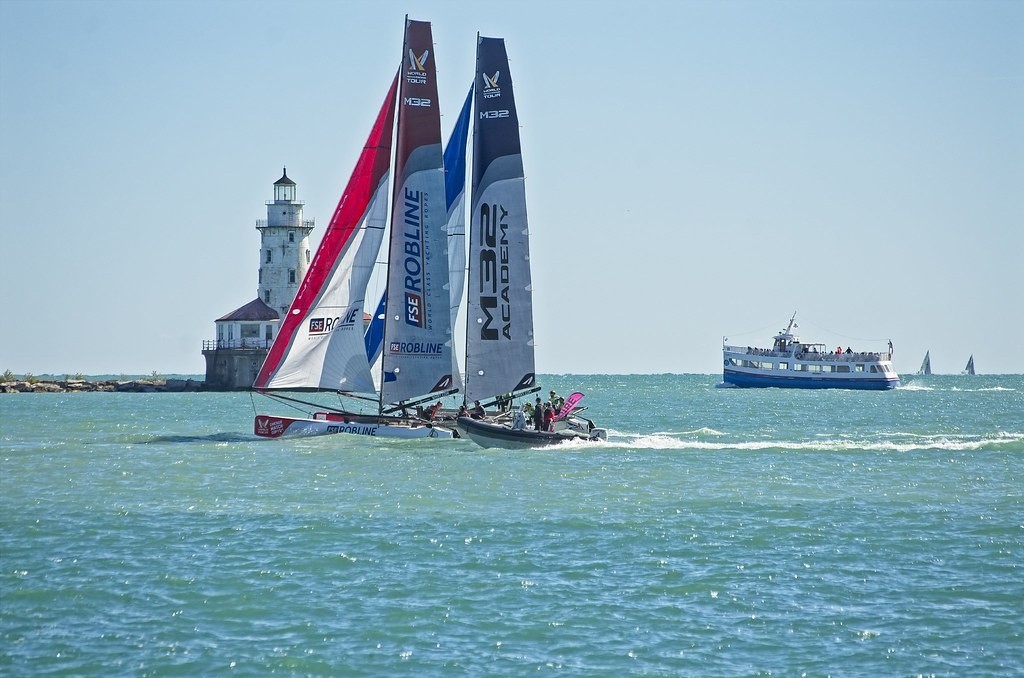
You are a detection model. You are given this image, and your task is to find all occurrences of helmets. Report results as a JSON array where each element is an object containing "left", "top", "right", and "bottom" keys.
[
  {"left": 459, "top": 406, "right": 466, "bottom": 411},
  {"left": 525, "top": 403, "right": 531, "bottom": 407},
  {"left": 550, "top": 390, "right": 556, "bottom": 394},
  {"left": 560, "top": 396, "right": 564, "bottom": 402},
  {"left": 474, "top": 400, "right": 481, "bottom": 405}
]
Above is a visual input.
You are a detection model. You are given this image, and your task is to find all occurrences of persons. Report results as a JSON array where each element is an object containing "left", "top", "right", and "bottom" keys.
[
  {"left": 454, "top": 405, "right": 471, "bottom": 419},
  {"left": 422, "top": 401, "right": 443, "bottom": 421},
  {"left": 471, "top": 400, "right": 486, "bottom": 419},
  {"left": 845, "top": 347, "right": 853, "bottom": 353},
  {"left": 524, "top": 390, "right": 565, "bottom": 432}
]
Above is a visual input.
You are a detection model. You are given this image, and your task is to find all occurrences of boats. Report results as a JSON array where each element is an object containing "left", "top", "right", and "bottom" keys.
[
  {"left": 166, "top": 379, "right": 186, "bottom": 391},
  {"left": 721, "top": 313, "right": 896, "bottom": 388}
]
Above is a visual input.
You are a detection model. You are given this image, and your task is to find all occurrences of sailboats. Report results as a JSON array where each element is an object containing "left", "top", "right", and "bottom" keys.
[
  {"left": 918, "top": 351, "right": 934, "bottom": 375},
  {"left": 963, "top": 356, "right": 977, "bottom": 375},
  {"left": 249, "top": 12, "right": 612, "bottom": 451}
]
